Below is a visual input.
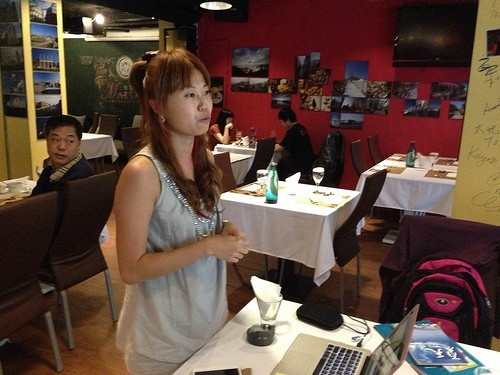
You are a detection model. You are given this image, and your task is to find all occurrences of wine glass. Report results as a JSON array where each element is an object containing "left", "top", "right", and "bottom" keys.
[
  {"left": 429, "top": 152, "right": 439, "bottom": 171},
  {"left": 257, "top": 169, "right": 268, "bottom": 193},
  {"left": 312, "top": 167, "right": 324, "bottom": 194},
  {"left": 236, "top": 131, "right": 242, "bottom": 146}
]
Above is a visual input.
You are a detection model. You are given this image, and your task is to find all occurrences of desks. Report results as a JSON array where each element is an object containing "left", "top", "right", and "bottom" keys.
[
  {"left": 212, "top": 151, "right": 253, "bottom": 185},
  {"left": 214, "top": 143, "right": 289, "bottom": 160},
  {"left": 171, "top": 297, "right": 500, "bottom": 375},
  {"left": 221, "top": 180, "right": 365, "bottom": 302},
  {"left": 81, "top": 133, "right": 118, "bottom": 173},
  {"left": 355, "top": 153, "right": 458, "bottom": 244}
]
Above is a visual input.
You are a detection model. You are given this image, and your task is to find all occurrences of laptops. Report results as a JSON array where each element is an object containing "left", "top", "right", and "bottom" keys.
[{"left": 271, "top": 304, "right": 420, "bottom": 375}]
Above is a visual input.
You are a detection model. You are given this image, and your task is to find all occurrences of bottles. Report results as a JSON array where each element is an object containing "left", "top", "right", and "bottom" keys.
[
  {"left": 406, "top": 141, "right": 416, "bottom": 167},
  {"left": 266, "top": 163, "right": 278, "bottom": 203},
  {"left": 249, "top": 127, "right": 256, "bottom": 148}
]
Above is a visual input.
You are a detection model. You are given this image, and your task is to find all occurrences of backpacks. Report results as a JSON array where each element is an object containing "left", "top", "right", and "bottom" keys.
[{"left": 399, "top": 257, "right": 493, "bottom": 349}]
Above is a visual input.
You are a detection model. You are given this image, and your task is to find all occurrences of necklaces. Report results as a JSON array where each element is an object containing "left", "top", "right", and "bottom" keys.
[{"left": 165, "top": 172, "right": 217, "bottom": 238}]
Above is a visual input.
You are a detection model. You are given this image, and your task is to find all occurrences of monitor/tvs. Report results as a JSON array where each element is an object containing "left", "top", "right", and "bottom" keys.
[{"left": 393, "top": 4, "right": 479, "bottom": 67}]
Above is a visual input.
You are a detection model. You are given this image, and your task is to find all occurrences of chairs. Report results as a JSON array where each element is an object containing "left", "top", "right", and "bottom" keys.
[
  {"left": 212, "top": 132, "right": 500, "bottom": 350},
  {"left": 0, "top": 112, "right": 145, "bottom": 372}
]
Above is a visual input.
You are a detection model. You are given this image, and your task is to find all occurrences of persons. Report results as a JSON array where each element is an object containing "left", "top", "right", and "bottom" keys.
[
  {"left": 114, "top": 48, "right": 248, "bottom": 375},
  {"left": 275, "top": 107, "right": 313, "bottom": 180},
  {"left": 205, "top": 108, "right": 233, "bottom": 150},
  {"left": 29, "top": 115, "right": 96, "bottom": 270}
]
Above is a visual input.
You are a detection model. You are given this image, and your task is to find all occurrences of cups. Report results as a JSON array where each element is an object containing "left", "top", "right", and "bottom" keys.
[
  {"left": 8, "top": 182, "right": 22, "bottom": 192},
  {"left": 257, "top": 293, "right": 282, "bottom": 329},
  {"left": 418, "top": 156, "right": 425, "bottom": 168},
  {"left": 241, "top": 136, "right": 249, "bottom": 146}
]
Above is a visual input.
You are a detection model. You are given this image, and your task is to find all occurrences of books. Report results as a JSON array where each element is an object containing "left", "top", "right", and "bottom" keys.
[{"left": 375, "top": 319, "right": 491, "bottom": 375}]
